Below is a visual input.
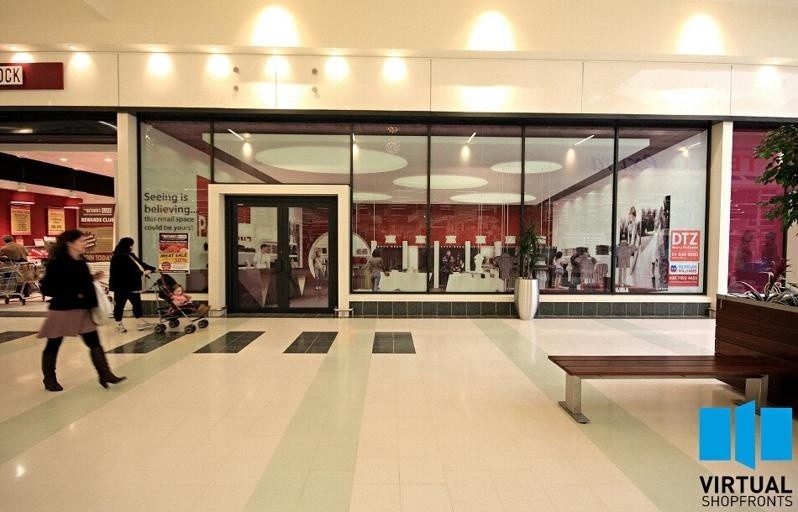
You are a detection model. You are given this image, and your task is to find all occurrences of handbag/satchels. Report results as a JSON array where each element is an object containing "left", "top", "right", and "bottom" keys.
[{"left": 86, "top": 263, "right": 114, "bottom": 326}]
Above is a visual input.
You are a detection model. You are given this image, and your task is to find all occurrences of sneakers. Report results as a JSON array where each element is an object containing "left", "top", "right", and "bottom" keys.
[
  {"left": 114, "top": 323, "right": 127, "bottom": 333},
  {"left": 136, "top": 322, "right": 154, "bottom": 330}
]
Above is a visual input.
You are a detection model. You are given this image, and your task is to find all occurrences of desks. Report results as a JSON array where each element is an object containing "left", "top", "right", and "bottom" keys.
[
  {"left": 443, "top": 272, "right": 507, "bottom": 294},
  {"left": 378, "top": 271, "right": 433, "bottom": 293}
]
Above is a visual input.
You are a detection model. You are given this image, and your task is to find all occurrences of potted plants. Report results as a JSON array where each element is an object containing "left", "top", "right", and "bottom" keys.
[{"left": 512, "top": 220, "right": 546, "bottom": 322}]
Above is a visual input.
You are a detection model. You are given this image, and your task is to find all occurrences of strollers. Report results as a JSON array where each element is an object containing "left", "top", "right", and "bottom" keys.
[{"left": 141, "top": 268, "right": 210, "bottom": 334}]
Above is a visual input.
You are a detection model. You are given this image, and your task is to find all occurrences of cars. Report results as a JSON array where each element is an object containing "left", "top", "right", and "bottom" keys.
[{"left": 731, "top": 259, "right": 778, "bottom": 286}]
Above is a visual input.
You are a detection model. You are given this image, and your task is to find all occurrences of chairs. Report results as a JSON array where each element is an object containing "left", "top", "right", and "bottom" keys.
[
  {"left": 493, "top": 252, "right": 519, "bottom": 285},
  {"left": 570, "top": 254, "right": 610, "bottom": 290}
]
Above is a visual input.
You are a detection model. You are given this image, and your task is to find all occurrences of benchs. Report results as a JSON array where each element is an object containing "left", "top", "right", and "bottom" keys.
[{"left": 547, "top": 353, "right": 789, "bottom": 426}]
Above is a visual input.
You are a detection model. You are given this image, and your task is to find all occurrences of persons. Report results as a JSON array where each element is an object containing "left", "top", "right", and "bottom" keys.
[
  {"left": 616, "top": 241, "right": 637, "bottom": 267},
  {"left": 574, "top": 251, "right": 597, "bottom": 275},
  {"left": 0, "top": 234, "right": 32, "bottom": 297},
  {"left": 109, "top": 238, "right": 159, "bottom": 334},
  {"left": 760, "top": 231, "right": 778, "bottom": 262},
  {"left": 37, "top": 229, "right": 127, "bottom": 391},
  {"left": 314, "top": 250, "right": 323, "bottom": 290},
  {"left": 456, "top": 255, "right": 465, "bottom": 271},
  {"left": 171, "top": 284, "right": 211, "bottom": 317},
  {"left": 624, "top": 206, "right": 667, "bottom": 245},
  {"left": 367, "top": 248, "right": 387, "bottom": 294},
  {"left": 553, "top": 251, "right": 564, "bottom": 289},
  {"left": 442, "top": 249, "right": 455, "bottom": 288},
  {"left": 253, "top": 244, "right": 270, "bottom": 270},
  {"left": 734, "top": 230, "right": 754, "bottom": 292}
]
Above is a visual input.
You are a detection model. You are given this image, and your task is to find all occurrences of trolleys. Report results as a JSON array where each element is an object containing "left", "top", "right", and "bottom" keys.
[{"left": 1, "top": 257, "right": 49, "bottom": 305}]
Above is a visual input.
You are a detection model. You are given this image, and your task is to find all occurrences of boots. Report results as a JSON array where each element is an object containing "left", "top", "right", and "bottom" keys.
[
  {"left": 42, "top": 351, "right": 63, "bottom": 391},
  {"left": 91, "top": 344, "right": 126, "bottom": 389}
]
